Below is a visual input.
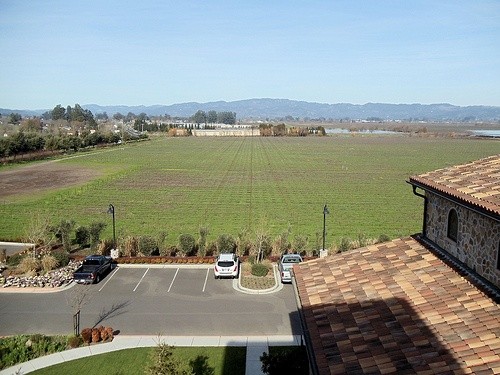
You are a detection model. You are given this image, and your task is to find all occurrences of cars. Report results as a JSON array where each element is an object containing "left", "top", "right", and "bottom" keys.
[{"left": 213, "top": 253, "right": 240, "bottom": 279}]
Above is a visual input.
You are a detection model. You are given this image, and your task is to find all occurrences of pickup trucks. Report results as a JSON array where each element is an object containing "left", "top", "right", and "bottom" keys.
[
  {"left": 278, "top": 254, "right": 303, "bottom": 283},
  {"left": 73, "top": 254, "right": 114, "bottom": 284}
]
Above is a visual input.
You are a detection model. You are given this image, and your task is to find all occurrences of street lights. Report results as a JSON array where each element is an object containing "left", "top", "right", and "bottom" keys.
[
  {"left": 106, "top": 203, "right": 117, "bottom": 250},
  {"left": 322, "top": 205, "right": 331, "bottom": 251}
]
